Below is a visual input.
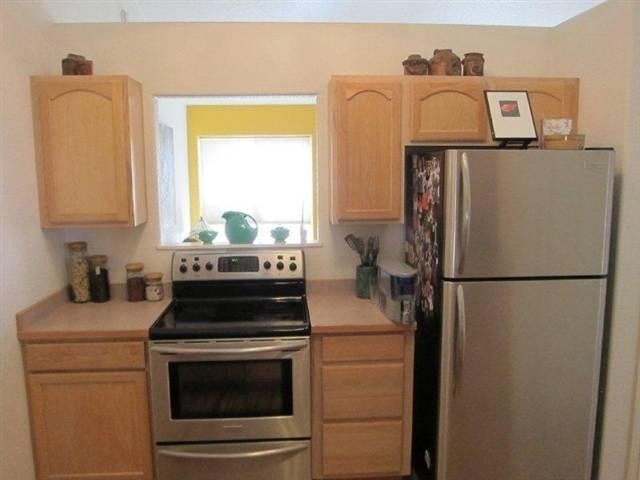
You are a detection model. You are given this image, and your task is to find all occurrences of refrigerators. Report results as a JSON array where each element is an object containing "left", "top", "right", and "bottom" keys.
[{"left": 404, "top": 146, "right": 618, "bottom": 478}]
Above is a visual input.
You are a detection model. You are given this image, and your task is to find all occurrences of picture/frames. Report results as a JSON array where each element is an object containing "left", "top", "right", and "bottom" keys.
[{"left": 484, "top": 90, "right": 538, "bottom": 141}]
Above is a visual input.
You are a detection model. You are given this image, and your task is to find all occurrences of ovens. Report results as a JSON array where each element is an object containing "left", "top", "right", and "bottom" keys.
[{"left": 147, "top": 334, "right": 317, "bottom": 479}]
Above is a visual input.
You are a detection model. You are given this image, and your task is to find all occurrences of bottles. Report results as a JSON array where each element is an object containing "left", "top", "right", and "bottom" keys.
[
  {"left": 87, "top": 255, "right": 110, "bottom": 301},
  {"left": 126, "top": 263, "right": 145, "bottom": 302},
  {"left": 143, "top": 273, "right": 163, "bottom": 301},
  {"left": 69, "top": 242, "right": 91, "bottom": 303}
]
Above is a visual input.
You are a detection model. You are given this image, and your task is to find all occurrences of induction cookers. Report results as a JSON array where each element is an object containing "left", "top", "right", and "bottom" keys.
[{"left": 149, "top": 249, "right": 314, "bottom": 340}]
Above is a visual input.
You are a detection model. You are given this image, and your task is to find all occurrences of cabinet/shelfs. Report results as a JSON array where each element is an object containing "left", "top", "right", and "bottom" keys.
[
  {"left": 406, "top": 78, "right": 580, "bottom": 146},
  {"left": 30, "top": 76, "right": 148, "bottom": 229},
  {"left": 14, "top": 281, "right": 174, "bottom": 479},
  {"left": 328, "top": 74, "right": 405, "bottom": 226},
  {"left": 305, "top": 277, "right": 417, "bottom": 478}
]
